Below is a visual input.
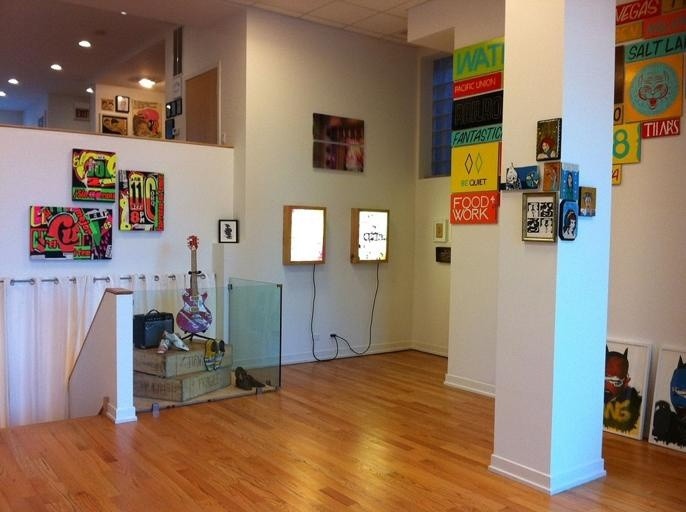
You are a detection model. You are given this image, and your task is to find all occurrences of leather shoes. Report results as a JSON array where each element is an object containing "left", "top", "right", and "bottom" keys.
[
  {"left": 215, "top": 340, "right": 225, "bottom": 369},
  {"left": 205, "top": 339, "right": 217, "bottom": 371},
  {"left": 157, "top": 337, "right": 169, "bottom": 354},
  {"left": 165, "top": 331, "right": 189, "bottom": 350}
]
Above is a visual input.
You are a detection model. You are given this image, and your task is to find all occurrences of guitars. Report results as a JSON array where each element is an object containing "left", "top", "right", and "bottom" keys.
[{"left": 176, "top": 235, "right": 212, "bottom": 334}]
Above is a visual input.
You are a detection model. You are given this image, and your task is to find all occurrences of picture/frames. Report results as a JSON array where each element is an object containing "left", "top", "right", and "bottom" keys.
[
  {"left": 523, "top": 192, "right": 556, "bottom": 243},
  {"left": 219, "top": 220, "right": 238, "bottom": 245},
  {"left": 649, "top": 346, "right": 686, "bottom": 452},
  {"left": 100, "top": 114, "right": 128, "bottom": 135},
  {"left": 165, "top": 119, "right": 175, "bottom": 139},
  {"left": 115, "top": 95, "right": 130, "bottom": 113},
  {"left": 99, "top": 99, "right": 114, "bottom": 112},
  {"left": 75, "top": 108, "right": 89, "bottom": 119},
  {"left": 167, "top": 99, "right": 182, "bottom": 118},
  {"left": 602, "top": 339, "right": 651, "bottom": 441},
  {"left": 536, "top": 119, "right": 563, "bottom": 161}
]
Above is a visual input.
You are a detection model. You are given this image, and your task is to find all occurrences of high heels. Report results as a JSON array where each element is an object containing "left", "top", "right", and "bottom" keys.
[
  {"left": 235, "top": 366, "right": 251, "bottom": 389},
  {"left": 237, "top": 374, "right": 264, "bottom": 387}
]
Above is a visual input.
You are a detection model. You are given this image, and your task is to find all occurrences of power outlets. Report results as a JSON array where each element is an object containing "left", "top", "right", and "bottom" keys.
[{"left": 330, "top": 331, "right": 338, "bottom": 340}]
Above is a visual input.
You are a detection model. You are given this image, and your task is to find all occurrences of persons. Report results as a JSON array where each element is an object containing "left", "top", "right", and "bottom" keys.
[
  {"left": 583, "top": 193, "right": 592, "bottom": 214},
  {"left": 536, "top": 139, "right": 556, "bottom": 160},
  {"left": 566, "top": 173, "right": 574, "bottom": 200},
  {"left": 563, "top": 210, "right": 577, "bottom": 239}
]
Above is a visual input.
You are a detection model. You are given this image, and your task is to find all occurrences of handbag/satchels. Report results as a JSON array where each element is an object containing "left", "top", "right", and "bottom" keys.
[{"left": 134, "top": 310, "right": 174, "bottom": 349}]
